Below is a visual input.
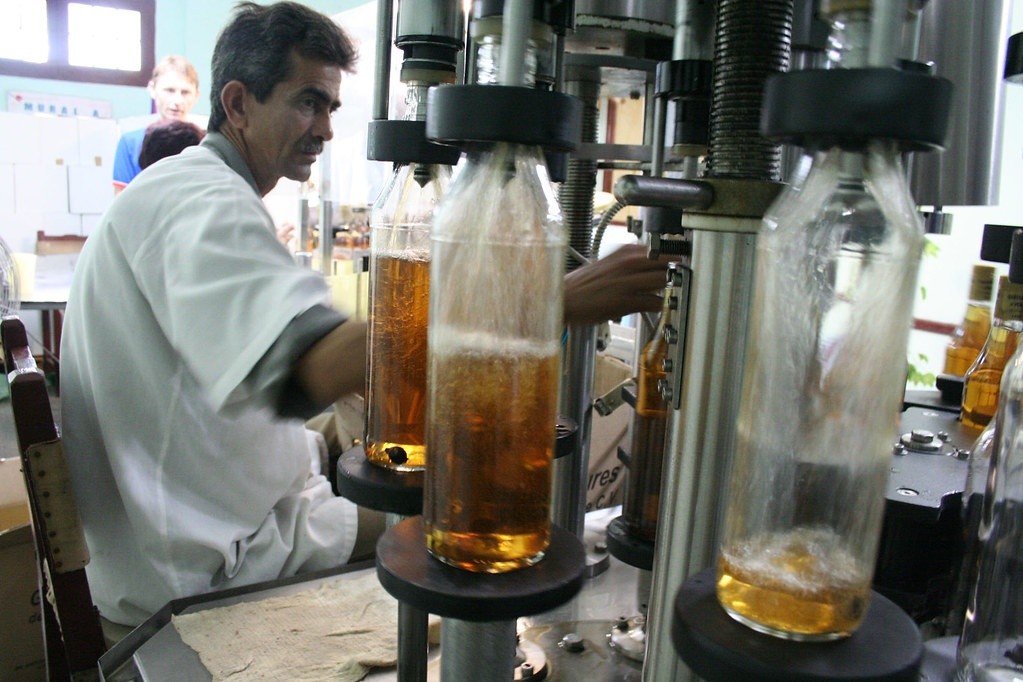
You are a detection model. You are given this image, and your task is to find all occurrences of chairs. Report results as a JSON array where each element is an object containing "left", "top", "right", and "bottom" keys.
[
  {"left": 0, "top": 315, "right": 108, "bottom": 682},
  {"left": 36, "top": 230, "right": 89, "bottom": 360}
]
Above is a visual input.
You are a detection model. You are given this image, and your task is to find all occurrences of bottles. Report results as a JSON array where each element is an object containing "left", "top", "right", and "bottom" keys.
[
  {"left": 941, "top": 265, "right": 1023, "bottom": 682},
  {"left": 369, "top": 43, "right": 459, "bottom": 473},
  {"left": 424, "top": 38, "right": 568, "bottom": 575},
  {"left": 718, "top": 8, "right": 923, "bottom": 642}
]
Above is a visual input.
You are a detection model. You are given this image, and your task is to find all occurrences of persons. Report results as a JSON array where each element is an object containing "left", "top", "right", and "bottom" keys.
[
  {"left": 112, "top": 54, "right": 209, "bottom": 203},
  {"left": 59, "top": 1, "right": 682, "bottom": 657}
]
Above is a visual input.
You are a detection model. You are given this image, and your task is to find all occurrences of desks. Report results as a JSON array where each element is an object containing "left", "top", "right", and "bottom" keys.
[{"left": 7, "top": 254, "right": 80, "bottom": 397}]
